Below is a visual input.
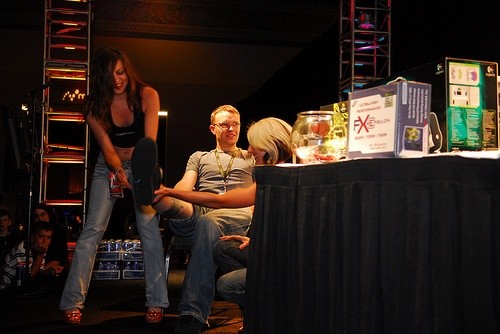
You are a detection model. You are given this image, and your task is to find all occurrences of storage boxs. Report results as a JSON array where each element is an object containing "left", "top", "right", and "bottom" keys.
[{"left": 346, "top": 81, "right": 432, "bottom": 159}]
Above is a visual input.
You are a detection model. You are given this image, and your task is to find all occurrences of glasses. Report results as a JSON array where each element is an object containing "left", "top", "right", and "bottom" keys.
[{"left": 212, "top": 121, "right": 241, "bottom": 129}]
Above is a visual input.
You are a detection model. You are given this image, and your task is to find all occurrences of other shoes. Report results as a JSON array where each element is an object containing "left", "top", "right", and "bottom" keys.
[
  {"left": 168, "top": 315, "right": 208, "bottom": 333},
  {"left": 131, "top": 135, "right": 161, "bottom": 206}
]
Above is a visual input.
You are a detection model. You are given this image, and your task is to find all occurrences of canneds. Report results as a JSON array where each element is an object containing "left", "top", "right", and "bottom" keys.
[{"left": 92, "top": 239, "right": 145, "bottom": 280}]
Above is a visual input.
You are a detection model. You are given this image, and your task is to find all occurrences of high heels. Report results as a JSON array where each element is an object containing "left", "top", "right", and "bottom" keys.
[
  {"left": 65, "top": 308, "right": 81, "bottom": 324},
  {"left": 146, "top": 306, "right": 163, "bottom": 323}
]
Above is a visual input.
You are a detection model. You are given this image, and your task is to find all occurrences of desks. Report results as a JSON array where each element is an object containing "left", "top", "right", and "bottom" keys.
[{"left": 243, "top": 156, "right": 500, "bottom": 334}]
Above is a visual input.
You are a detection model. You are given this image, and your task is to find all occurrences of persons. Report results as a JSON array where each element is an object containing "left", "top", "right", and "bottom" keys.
[
  {"left": 31, "top": 203, "right": 72, "bottom": 268},
  {"left": 150, "top": 116, "right": 302, "bottom": 334},
  {"left": 131, "top": 104, "right": 256, "bottom": 334},
  {"left": 59, "top": 43, "right": 169, "bottom": 324},
  {"left": 0, "top": 208, "right": 15, "bottom": 268},
  {"left": 0, "top": 220, "right": 59, "bottom": 292}
]
